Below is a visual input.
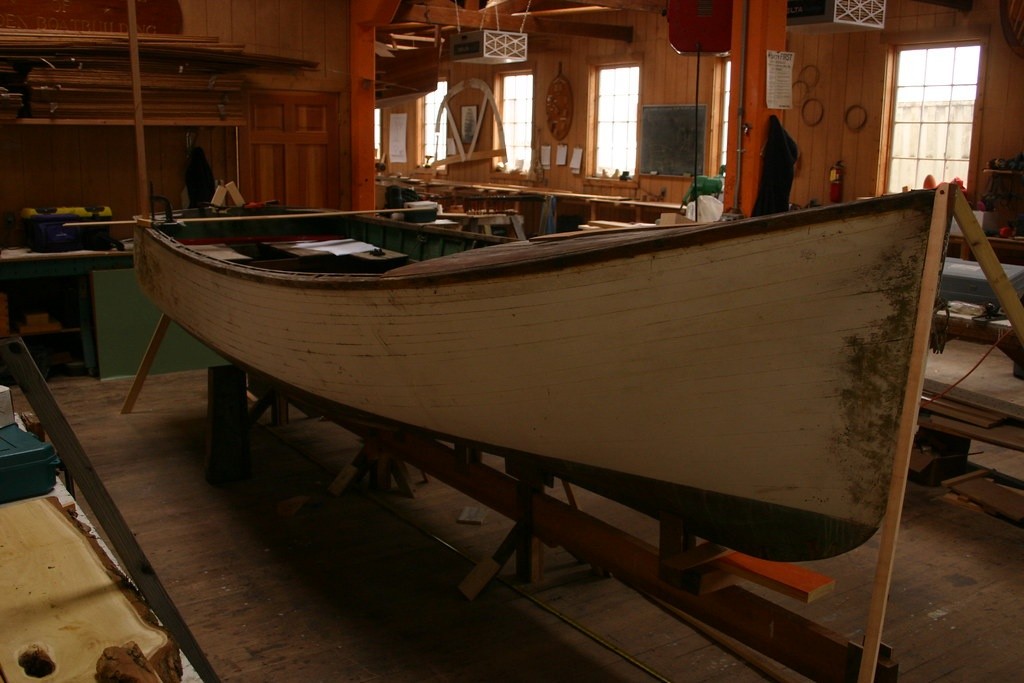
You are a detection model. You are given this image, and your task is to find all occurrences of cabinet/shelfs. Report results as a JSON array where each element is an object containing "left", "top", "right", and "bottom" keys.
[{"left": 2, "top": 253, "right": 98, "bottom": 379}]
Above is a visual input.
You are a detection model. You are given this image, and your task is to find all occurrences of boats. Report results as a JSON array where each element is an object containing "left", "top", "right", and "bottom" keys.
[{"left": 132, "top": 182, "right": 953, "bottom": 564}]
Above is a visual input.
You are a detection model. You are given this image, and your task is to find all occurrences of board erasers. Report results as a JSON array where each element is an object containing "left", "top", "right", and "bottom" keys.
[
  {"left": 650, "top": 171, "right": 659, "bottom": 175},
  {"left": 683, "top": 173, "right": 691, "bottom": 177}
]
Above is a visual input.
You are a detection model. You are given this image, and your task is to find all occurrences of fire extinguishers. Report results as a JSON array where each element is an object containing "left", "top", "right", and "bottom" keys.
[{"left": 829, "top": 159, "right": 845, "bottom": 203}]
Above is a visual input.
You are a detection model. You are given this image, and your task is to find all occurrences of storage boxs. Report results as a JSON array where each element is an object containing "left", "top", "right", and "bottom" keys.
[
  {"left": 983, "top": 476, "right": 1024, "bottom": 526},
  {"left": 24, "top": 412, "right": 45, "bottom": 441},
  {"left": 909, "top": 425, "right": 971, "bottom": 486},
  {"left": 0, "top": 385, "right": 16, "bottom": 429},
  {"left": 19, "top": 204, "right": 117, "bottom": 251}
]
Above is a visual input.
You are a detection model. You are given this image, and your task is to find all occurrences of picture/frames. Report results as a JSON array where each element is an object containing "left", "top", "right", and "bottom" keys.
[{"left": 460, "top": 104, "right": 478, "bottom": 144}]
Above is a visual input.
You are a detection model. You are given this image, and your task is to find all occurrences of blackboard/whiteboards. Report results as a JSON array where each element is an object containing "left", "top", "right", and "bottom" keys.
[{"left": 638, "top": 103, "right": 705, "bottom": 178}]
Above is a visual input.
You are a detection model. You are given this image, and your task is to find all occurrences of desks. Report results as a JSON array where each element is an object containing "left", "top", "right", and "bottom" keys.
[{"left": 422, "top": 178, "right": 689, "bottom": 224}]
